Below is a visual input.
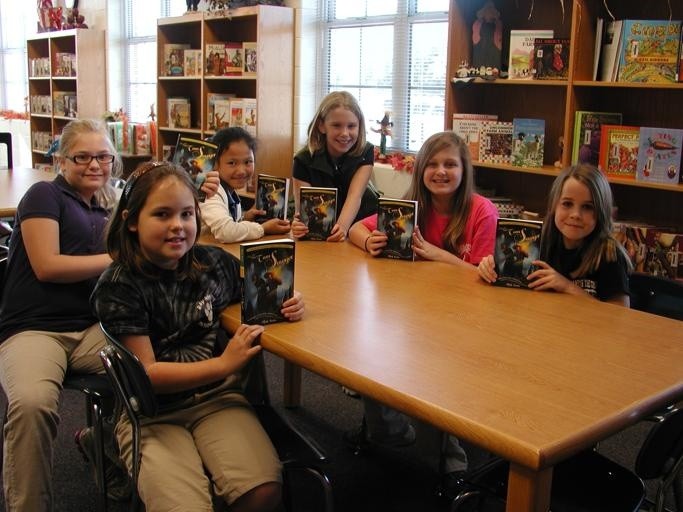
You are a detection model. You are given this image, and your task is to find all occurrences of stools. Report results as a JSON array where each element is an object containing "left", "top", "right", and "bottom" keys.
[{"left": 54, "top": 369, "right": 113, "bottom": 512}]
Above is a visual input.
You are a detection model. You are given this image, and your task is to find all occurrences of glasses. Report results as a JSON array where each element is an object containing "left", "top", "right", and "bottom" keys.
[{"left": 62, "top": 150, "right": 115, "bottom": 165}]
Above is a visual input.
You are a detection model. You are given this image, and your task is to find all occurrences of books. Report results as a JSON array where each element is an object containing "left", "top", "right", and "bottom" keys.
[
  {"left": 376, "top": 198, "right": 418, "bottom": 263},
  {"left": 238, "top": 239, "right": 296, "bottom": 326},
  {"left": 106, "top": 122, "right": 156, "bottom": 156},
  {"left": 163, "top": 41, "right": 258, "bottom": 203},
  {"left": 256, "top": 173, "right": 289, "bottom": 224},
  {"left": 298, "top": 186, "right": 338, "bottom": 241},
  {"left": 29, "top": 51, "right": 77, "bottom": 173}
]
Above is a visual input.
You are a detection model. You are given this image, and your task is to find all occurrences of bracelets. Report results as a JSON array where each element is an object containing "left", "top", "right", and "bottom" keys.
[{"left": 365, "top": 235, "right": 370, "bottom": 252}]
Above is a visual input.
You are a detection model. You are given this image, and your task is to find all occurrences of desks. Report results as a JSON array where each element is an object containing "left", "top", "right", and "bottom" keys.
[
  {"left": 0, "top": 163, "right": 57, "bottom": 222},
  {"left": 115, "top": 149, "right": 149, "bottom": 180},
  {"left": 187, "top": 231, "right": 682, "bottom": 510}
]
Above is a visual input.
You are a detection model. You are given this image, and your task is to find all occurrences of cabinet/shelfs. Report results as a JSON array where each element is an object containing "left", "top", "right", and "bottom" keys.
[
  {"left": 28, "top": 29, "right": 106, "bottom": 173},
  {"left": 440, "top": 0, "right": 682, "bottom": 279},
  {"left": 154, "top": 4, "right": 295, "bottom": 196}
]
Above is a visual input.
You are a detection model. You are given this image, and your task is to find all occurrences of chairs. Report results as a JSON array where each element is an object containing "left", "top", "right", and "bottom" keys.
[
  {"left": 98, "top": 318, "right": 335, "bottom": 510},
  {"left": 434, "top": 390, "right": 683, "bottom": 511},
  {"left": 0, "top": 130, "right": 13, "bottom": 252}
]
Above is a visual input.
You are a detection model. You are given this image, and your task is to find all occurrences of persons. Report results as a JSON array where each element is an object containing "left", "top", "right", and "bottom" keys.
[
  {"left": 196, "top": 127, "right": 290, "bottom": 245},
  {"left": 475, "top": 162, "right": 633, "bottom": 308},
  {"left": 291, "top": 91, "right": 380, "bottom": 395},
  {"left": 349, "top": 132, "right": 499, "bottom": 494},
  {"left": 0, "top": 119, "right": 124, "bottom": 511},
  {"left": 88, "top": 161, "right": 305, "bottom": 511}
]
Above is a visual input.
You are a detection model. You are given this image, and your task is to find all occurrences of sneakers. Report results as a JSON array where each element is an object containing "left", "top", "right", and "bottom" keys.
[
  {"left": 341, "top": 425, "right": 367, "bottom": 451},
  {"left": 382, "top": 418, "right": 418, "bottom": 447},
  {"left": 435, "top": 443, "right": 469, "bottom": 475},
  {"left": 74, "top": 424, "right": 133, "bottom": 503},
  {"left": 338, "top": 385, "right": 362, "bottom": 399}
]
